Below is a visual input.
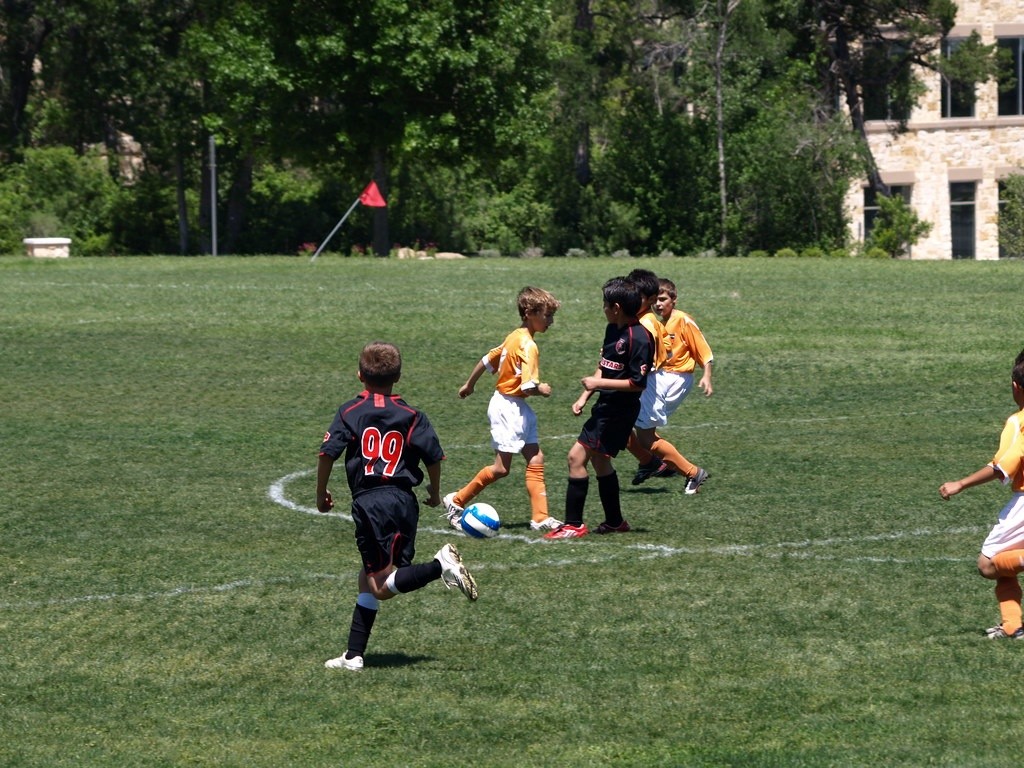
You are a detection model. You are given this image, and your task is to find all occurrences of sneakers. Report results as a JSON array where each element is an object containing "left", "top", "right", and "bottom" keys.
[
  {"left": 983, "top": 622, "right": 1024, "bottom": 640},
  {"left": 433, "top": 543, "right": 478, "bottom": 601},
  {"left": 325, "top": 650, "right": 364, "bottom": 672},
  {"left": 632, "top": 453, "right": 663, "bottom": 486},
  {"left": 443, "top": 492, "right": 465, "bottom": 532},
  {"left": 592, "top": 520, "right": 630, "bottom": 535},
  {"left": 652, "top": 463, "right": 676, "bottom": 477},
  {"left": 684, "top": 468, "right": 708, "bottom": 496},
  {"left": 545, "top": 524, "right": 590, "bottom": 539},
  {"left": 529, "top": 516, "right": 564, "bottom": 529}
]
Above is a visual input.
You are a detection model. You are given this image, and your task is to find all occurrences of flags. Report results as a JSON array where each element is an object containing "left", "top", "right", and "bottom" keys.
[{"left": 359, "top": 180, "right": 387, "bottom": 206}]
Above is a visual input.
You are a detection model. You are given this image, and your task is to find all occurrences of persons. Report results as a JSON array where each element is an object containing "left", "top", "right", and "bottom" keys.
[
  {"left": 545, "top": 277, "right": 655, "bottom": 540},
  {"left": 940, "top": 349, "right": 1024, "bottom": 641},
  {"left": 626, "top": 268, "right": 710, "bottom": 494},
  {"left": 637, "top": 279, "right": 713, "bottom": 474},
  {"left": 317, "top": 342, "right": 479, "bottom": 672},
  {"left": 443, "top": 286, "right": 565, "bottom": 532}
]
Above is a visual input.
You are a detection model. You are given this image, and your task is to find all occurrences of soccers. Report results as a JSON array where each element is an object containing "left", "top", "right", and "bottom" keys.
[{"left": 461, "top": 503, "right": 500, "bottom": 538}]
